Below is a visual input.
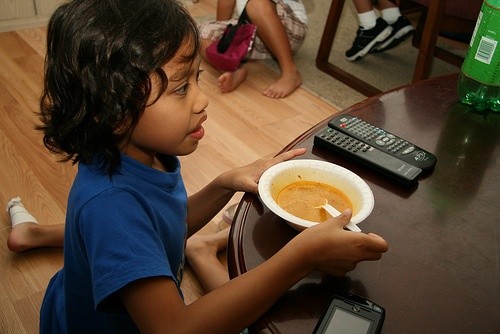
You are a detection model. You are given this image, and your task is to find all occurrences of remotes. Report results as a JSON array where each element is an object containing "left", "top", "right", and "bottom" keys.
[
  {"left": 313, "top": 127, "right": 423, "bottom": 184},
  {"left": 327, "top": 114, "right": 439, "bottom": 173}
]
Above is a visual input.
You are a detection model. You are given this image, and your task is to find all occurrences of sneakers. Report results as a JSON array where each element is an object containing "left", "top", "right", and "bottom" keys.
[
  {"left": 370, "top": 14, "right": 416, "bottom": 53},
  {"left": 344, "top": 17, "right": 394, "bottom": 62}
]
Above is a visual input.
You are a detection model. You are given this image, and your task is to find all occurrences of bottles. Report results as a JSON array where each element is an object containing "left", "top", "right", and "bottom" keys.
[{"left": 455, "top": 0, "right": 500, "bottom": 112}]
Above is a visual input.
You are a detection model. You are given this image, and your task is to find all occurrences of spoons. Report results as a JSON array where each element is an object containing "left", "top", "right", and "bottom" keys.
[{"left": 314, "top": 198, "right": 362, "bottom": 233}]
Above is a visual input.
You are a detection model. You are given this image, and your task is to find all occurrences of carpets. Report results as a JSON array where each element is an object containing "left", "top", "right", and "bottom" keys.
[{"left": 194, "top": 0, "right": 470, "bottom": 111}]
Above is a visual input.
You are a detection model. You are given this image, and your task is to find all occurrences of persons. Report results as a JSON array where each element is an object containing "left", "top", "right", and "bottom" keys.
[
  {"left": 346, "top": 0, "right": 416, "bottom": 60},
  {"left": 199, "top": 0, "right": 307, "bottom": 97},
  {"left": 7, "top": 0, "right": 387, "bottom": 333}
]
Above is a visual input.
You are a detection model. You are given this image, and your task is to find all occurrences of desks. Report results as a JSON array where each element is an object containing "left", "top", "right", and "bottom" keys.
[{"left": 225, "top": 73, "right": 500, "bottom": 334}]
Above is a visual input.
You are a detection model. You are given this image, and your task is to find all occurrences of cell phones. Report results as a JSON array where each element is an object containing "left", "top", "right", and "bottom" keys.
[{"left": 312, "top": 289, "right": 385, "bottom": 334}]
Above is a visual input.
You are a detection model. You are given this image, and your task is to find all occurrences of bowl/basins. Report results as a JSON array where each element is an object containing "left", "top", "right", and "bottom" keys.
[{"left": 257, "top": 159, "right": 375, "bottom": 235}]
